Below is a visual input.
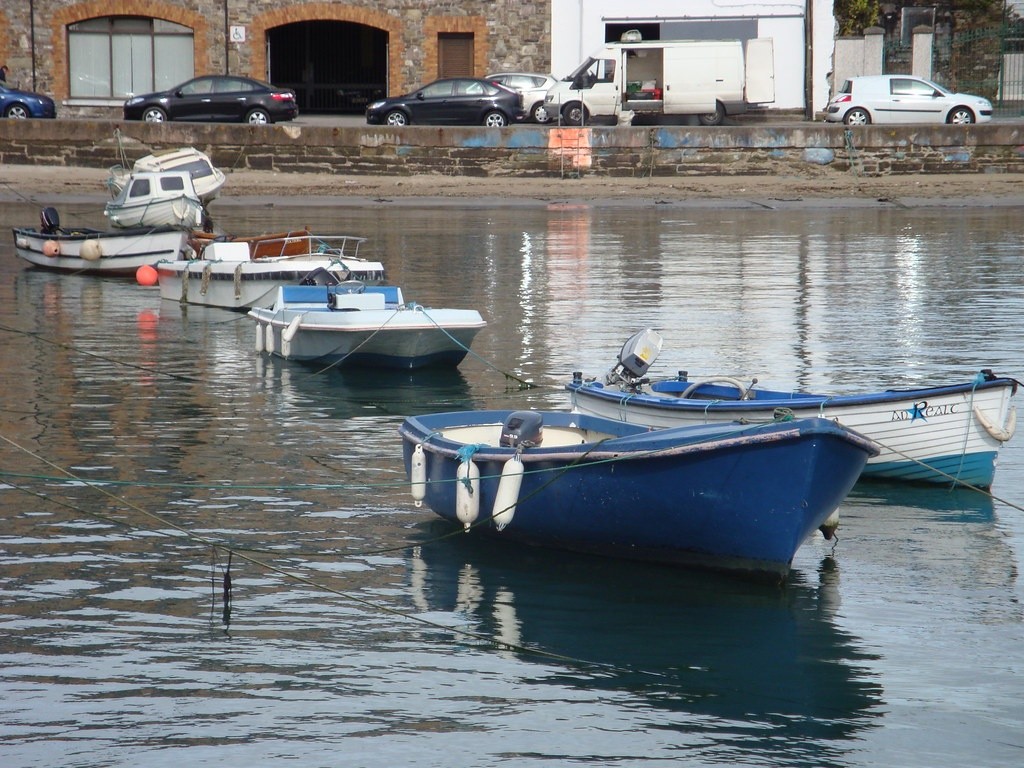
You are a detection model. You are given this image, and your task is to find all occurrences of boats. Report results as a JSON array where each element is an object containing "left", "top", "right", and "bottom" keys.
[
  {"left": 564, "top": 328, "right": 1017, "bottom": 488},
  {"left": 245, "top": 287, "right": 486, "bottom": 369},
  {"left": 155, "top": 231, "right": 386, "bottom": 318},
  {"left": 111, "top": 145, "right": 225, "bottom": 209},
  {"left": 106, "top": 170, "right": 212, "bottom": 233},
  {"left": 7, "top": 207, "right": 197, "bottom": 275},
  {"left": 400, "top": 402, "right": 874, "bottom": 589},
  {"left": 189, "top": 226, "right": 313, "bottom": 263}
]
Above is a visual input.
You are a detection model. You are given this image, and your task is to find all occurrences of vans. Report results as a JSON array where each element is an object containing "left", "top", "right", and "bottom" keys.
[{"left": 542, "top": 30, "right": 776, "bottom": 126}]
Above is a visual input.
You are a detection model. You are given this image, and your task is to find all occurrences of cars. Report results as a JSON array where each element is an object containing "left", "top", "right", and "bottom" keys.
[
  {"left": 1, "top": 80, "right": 56, "bottom": 121},
  {"left": 123, "top": 75, "right": 299, "bottom": 127},
  {"left": 464, "top": 73, "right": 560, "bottom": 126},
  {"left": 826, "top": 75, "right": 994, "bottom": 129},
  {"left": 364, "top": 77, "right": 524, "bottom": 133}
]
